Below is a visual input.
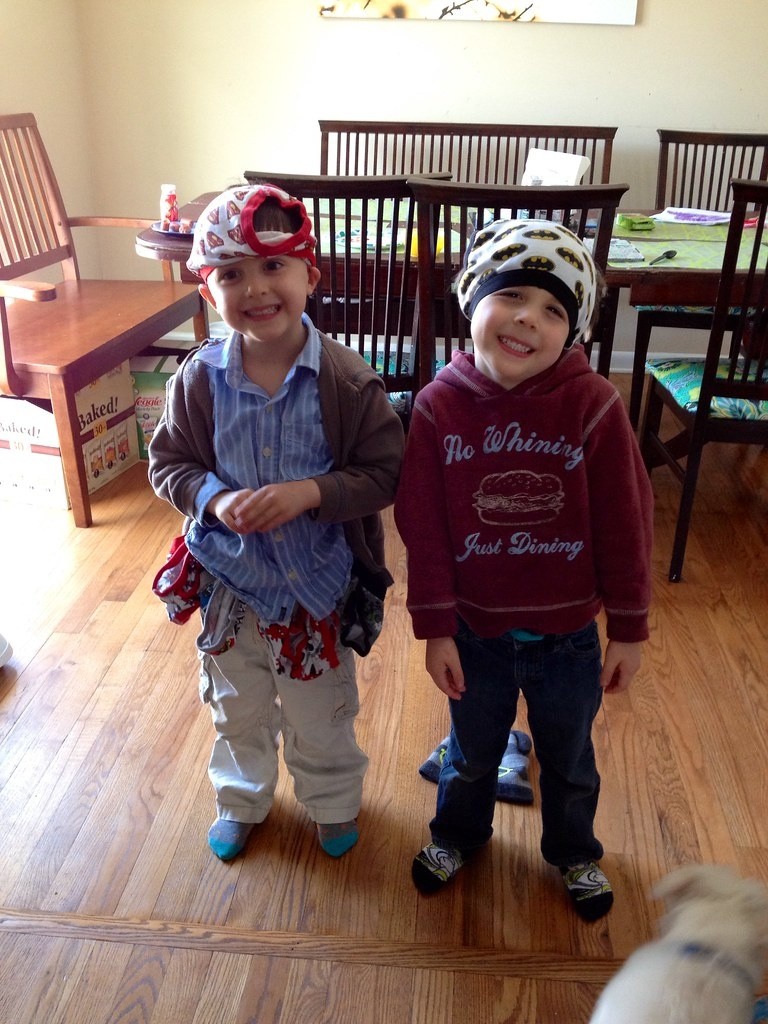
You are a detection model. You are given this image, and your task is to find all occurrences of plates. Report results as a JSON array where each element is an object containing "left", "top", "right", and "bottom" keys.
[
  {"left": 150, "top": 220, "right": 197, "bottom": 235},
  {"left": 335, "top": 228, "right": 404, "bottom": 250}
]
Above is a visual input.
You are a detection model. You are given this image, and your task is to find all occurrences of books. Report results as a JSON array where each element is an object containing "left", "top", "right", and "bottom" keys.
[{"left": 583, "top": 237, "right": 646, "bottom": 262}]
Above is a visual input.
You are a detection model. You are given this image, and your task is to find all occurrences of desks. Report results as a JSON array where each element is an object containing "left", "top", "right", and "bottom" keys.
[{"left": 132, "top": 189, "right": 768, "bottom": 342}]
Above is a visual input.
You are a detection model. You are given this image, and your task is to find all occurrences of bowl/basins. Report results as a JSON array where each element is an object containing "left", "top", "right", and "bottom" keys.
[{"left": 404, "top": 230, "right": 444, "bottom": 259}]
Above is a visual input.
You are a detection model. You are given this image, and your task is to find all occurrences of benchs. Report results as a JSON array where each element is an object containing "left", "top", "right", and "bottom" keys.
[{"left": 316, "top": 117, "right": 621, "bottom": 184}]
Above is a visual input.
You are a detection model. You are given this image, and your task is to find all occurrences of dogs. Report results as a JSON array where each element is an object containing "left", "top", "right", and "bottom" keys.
[{"left": 589, "top": 866, "right": 768, "bottom": 1024}]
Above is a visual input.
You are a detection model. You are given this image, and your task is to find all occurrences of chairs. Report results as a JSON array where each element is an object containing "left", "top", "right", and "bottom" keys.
[
  {"left": 404, "top": 179, "right": 628, "bottom": 399},
  {"left": 642, "top": 173, "right": 768, "bottom": 590},
  {"left": 629, "top": 129, "right": 768, "bottom": 427},
  {"left": 0, "top": 111, "right": 214, "bottom": 530},
  {"left": 239, "top": 165, "right": 453, "bottom": 418}
]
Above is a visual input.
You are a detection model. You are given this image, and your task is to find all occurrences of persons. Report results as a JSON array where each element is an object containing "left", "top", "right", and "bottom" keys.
[
  {"left": 147, "top": 186, "right": 406, "bottom": 863},
  {"left": 393, "top": 216, "right": 655, "bottom": 922}
]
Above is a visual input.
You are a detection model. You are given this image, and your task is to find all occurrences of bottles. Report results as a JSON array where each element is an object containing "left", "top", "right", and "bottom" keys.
[{"left": 160, "top": 183, "right": 178, "bottom": 231}]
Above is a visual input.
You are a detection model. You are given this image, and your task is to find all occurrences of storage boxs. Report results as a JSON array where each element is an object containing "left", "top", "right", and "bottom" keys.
[
  {"left": 130, "top": 331, "right": 204, "bottom": 464},
  {"left": 0, "top": 358, "right": 141, "bottom": 512}
]
